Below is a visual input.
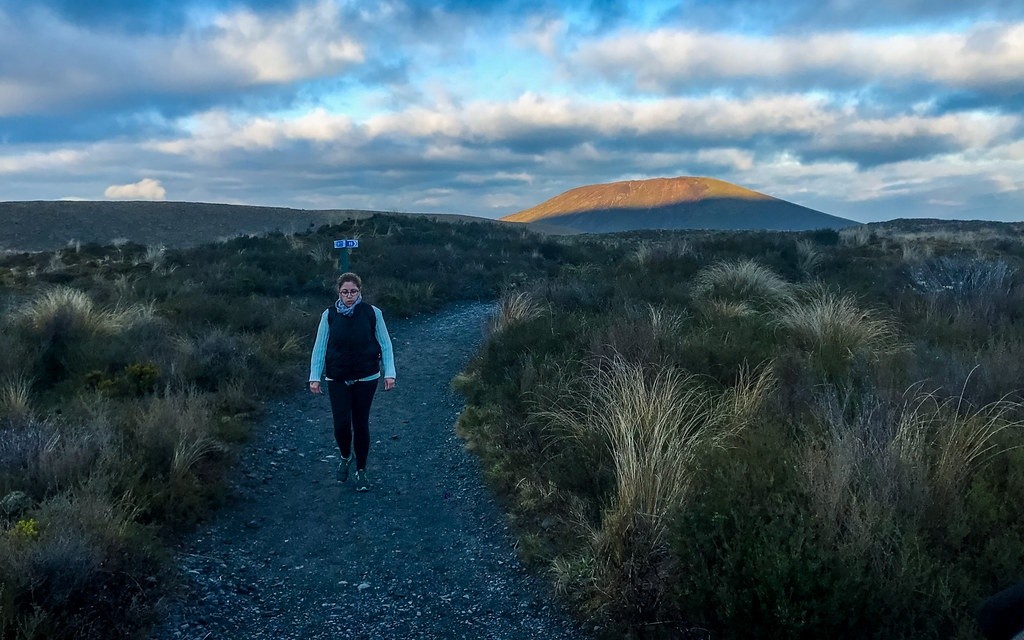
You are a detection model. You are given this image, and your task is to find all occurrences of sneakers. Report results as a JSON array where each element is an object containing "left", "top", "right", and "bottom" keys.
[
  {"left": 355, "top": 470, "right": 370, "bottom": 491},
  {"left": 337, "top": 453, "right": 354, "bottom": 482}
]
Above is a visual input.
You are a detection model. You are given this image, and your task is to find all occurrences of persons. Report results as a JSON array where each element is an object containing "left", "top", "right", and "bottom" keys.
[{"left": 308, "top": 271, "right": 397, "bottom": 492}]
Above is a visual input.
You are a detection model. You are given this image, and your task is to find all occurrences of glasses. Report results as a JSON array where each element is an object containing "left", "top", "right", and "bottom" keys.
[{"left": 339, "top": 289, "right": 360, "bottom": 297}]
